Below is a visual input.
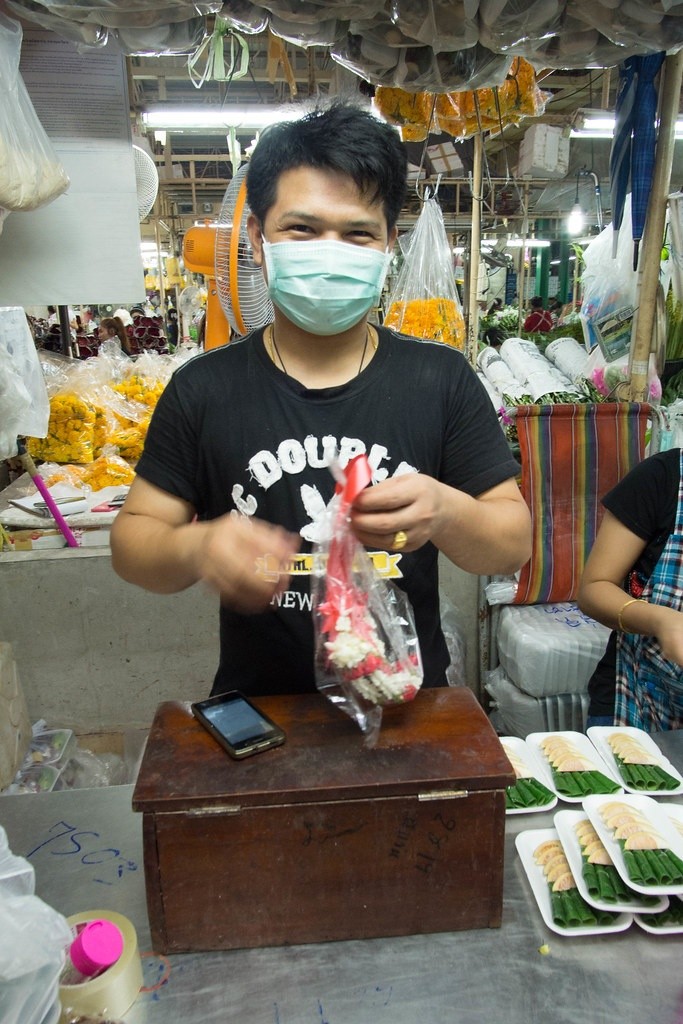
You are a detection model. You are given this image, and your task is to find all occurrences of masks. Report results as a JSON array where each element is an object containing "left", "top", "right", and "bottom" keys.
[{"left": 260, "top": 230, "right": 393, "bottom": 337}]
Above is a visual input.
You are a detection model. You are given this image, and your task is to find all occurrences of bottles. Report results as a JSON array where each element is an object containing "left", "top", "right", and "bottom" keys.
[{"left": 57, "top": 919, "right": 124, "bottom": 987}]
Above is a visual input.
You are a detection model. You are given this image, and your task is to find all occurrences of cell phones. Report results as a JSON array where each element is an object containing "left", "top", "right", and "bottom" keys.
[{"left": 190, "top": 690, "right": 286, "bottom": 760}]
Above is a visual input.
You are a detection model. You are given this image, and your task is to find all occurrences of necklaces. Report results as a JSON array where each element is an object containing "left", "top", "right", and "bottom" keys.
[{"left": 269, "top": 323, "right": 377, "bottom": 376}]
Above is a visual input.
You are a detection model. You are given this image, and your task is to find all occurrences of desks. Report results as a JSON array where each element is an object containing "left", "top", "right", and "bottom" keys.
[{"left": 0, "top": 728, "right": 683, "bottom": 1024}]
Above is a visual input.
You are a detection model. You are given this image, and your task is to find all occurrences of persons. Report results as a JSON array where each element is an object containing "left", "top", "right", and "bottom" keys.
[
  {"left": 548, "top": 297, "right": 563, "bottom": 323},
  {"left": 114, "top": 306, "right": 145, "bottom": 326},
  {"left": 524, "top": 296, "right": 552, "bottom": 332},
  {"left": 98, "top": 317, "right": 129, "bottom": 357},
  {"left": 577, "top": 448, "right": 683, "bottom": 733},
  {"left": 166, "top": 308, "right": 178, "bottom": 346},
  {"left": 487, "top": 298, "right": 502, "bottom": 347},
  {"left": 48, "top": 305, "right": 97, "bottom": 342},
  {"left": 110, "top": 103, "right": 532, "bottom": 699}
]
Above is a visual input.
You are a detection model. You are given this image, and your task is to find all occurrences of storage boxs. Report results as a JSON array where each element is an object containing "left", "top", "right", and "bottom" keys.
[
  {"left": 131, "top": 682, "right": 517, "bottom": 957},
  {"left": 0, "top": 641, "right": 34, "bottom": 788},
  {"left": 424, "top": 140, "right": 465, "bottom": 178},
  {"left": 518, "top": 124, "right": 570, "bottom": 179}
]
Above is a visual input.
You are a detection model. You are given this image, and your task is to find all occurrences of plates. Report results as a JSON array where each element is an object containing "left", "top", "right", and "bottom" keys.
[
  {"left": 499, "top": 725, "right": 683, "bottom": 938},
  {"left": 496, "top": 600, "right": 611, "bottom": 739}
]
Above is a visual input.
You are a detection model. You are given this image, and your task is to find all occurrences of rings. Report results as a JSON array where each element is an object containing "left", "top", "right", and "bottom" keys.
[{"left": 392, "top": 531, "right": 407, "bottom": 549}]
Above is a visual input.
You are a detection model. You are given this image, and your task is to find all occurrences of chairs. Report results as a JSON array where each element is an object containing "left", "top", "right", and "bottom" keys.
[{"left": 499, "top": 401, "right": 664, "bottom": 604}]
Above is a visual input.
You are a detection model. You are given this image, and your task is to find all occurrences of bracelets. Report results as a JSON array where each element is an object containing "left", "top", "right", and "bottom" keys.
[{"left": 618, "top": 600, "right": 648, "bottom": 634}]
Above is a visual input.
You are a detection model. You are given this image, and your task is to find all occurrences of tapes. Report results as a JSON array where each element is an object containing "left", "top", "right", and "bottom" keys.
[{"left": 56, "top": 909, "right": 143, "bottom": 1021}]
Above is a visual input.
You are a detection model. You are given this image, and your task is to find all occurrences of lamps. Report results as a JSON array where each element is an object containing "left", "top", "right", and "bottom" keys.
[
  {"left": 136, "top": 98, "right": 385, "bottom": 132},
  {"left": 454, "top": 238, "right": 551, "bottom": 256},
  {"left": 564, "top": 168, "right": 589, "bottom": 237},
  {"left": 570, "top": 108, "right": 683, "bottom": 140},
  {"left": 577, "top": 235, "right": 598, "bottom": 245}
]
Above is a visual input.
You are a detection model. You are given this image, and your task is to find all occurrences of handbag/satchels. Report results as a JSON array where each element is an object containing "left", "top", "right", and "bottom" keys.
[
  {"left": 380, "top": 199, "right": 467, "bottom": 354},
  {"left": 309, "top": 453, "right": 426, "bottom": 751}
]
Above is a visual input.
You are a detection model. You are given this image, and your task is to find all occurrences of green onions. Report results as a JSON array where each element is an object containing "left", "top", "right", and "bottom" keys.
[
  {"left": 548, "top": 828, "right": 683, "bottom": 930},
  {"left": 504, "top": 753, "right": 680, "bottom": 809}
]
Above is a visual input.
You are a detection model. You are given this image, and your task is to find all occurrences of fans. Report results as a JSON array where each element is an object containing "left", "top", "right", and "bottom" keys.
[
  {"left": 133, "top": 144, "right": 159, "bottom": 226},
  {"left": 181, "top": 164, "right": 275, "bottom": 355}
]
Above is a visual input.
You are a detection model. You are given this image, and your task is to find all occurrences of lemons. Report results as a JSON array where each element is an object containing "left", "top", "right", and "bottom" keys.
[
  {"left": 533, "top": 801, "right": 683, "bottom": 892},
  {"left": 500, "top": 732, "right": 660, "bottom": 778}
]
